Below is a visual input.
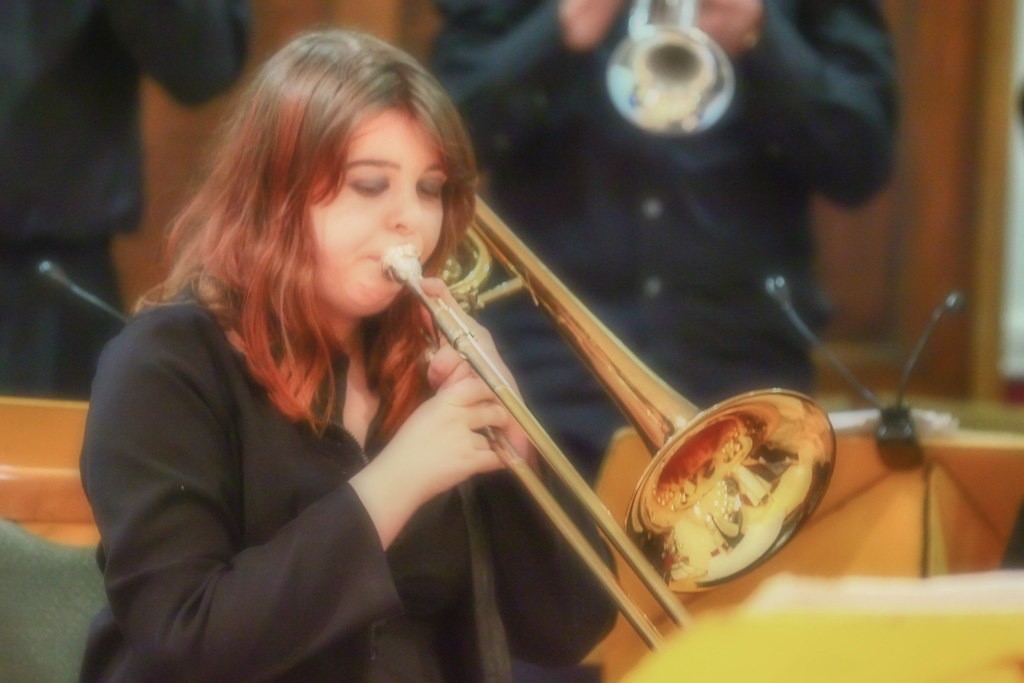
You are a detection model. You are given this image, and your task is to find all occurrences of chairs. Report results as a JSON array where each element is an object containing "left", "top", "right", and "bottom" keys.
[{"left": 0, "top": 520, "right": 105, "bottom": 683}]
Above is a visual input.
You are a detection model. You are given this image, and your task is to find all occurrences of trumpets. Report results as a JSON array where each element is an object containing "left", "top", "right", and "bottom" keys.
[{"left": 602, "top": 0, "right": 738, "bottom": 138}]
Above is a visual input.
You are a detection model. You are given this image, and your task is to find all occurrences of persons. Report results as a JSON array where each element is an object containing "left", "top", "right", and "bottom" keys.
[
  {"left": 77, "top": 29, "right": 620, "bottom": 683},
  {"left": 427, "top": 0, "right": 899, "bottom": 490},
  {"left": 0, "top": 0, "right": 253, "bottom": 403}
]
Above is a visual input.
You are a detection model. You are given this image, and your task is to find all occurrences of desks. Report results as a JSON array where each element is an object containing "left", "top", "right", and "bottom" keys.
[{"left": 574, "top": 423, "right": 1024, "bottom": 683}]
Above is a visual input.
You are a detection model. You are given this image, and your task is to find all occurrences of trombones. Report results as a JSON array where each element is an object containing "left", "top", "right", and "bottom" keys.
[{"left": 378, "top": 189, "right": 838, "bottom": 657}]
